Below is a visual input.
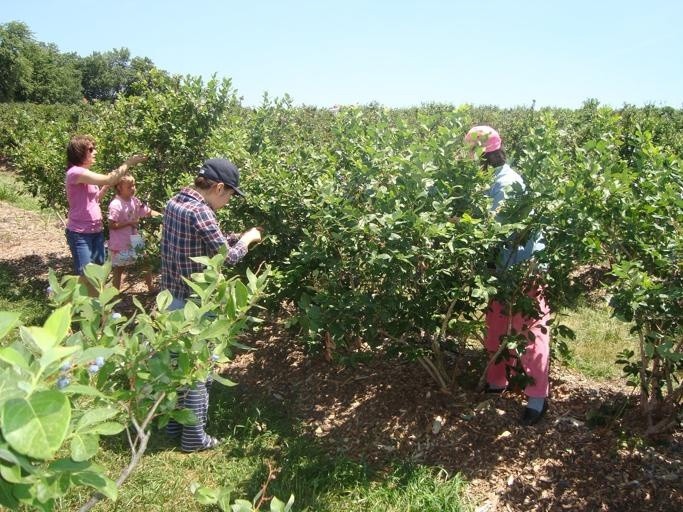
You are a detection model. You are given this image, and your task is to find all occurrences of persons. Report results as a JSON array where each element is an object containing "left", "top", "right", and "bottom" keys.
[
  {"left": 162, "top": 157, "right": 263, "bottom": 453},
  {"left": 63, "top": 136, "right": 161, "bottom": 326},
  {"left": 462, "top": 125, "right": 554, "bottom": 426}
]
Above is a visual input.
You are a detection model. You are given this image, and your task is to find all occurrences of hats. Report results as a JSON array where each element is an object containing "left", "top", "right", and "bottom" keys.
[
  {"left": 462, "top": 125, "right": 502, "bottom": 153},
  {"left": 198, "top": 157, "right": 247, "bottom": 198}
]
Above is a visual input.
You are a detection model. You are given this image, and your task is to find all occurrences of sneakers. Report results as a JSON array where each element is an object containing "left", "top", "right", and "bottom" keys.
[{"left": 518, "top": 398, "right": 548, "bottom": 427}]
